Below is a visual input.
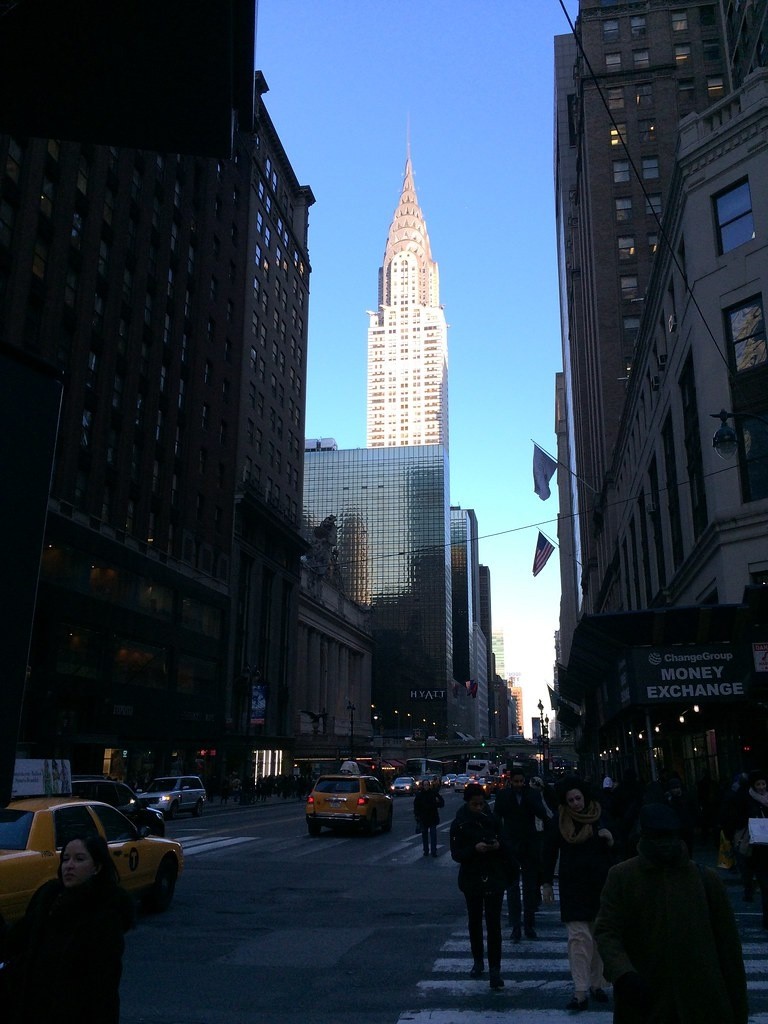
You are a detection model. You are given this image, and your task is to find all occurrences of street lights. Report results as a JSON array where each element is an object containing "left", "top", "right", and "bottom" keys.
[
  {"left": 538, "top": 700, "right": 549, "bottom": 775},
  {"left": 395, "top": 710, "right": 436, "bottom": 757},
  {"left": 370, "top": 704, "right": 381, "bottom": 778}
]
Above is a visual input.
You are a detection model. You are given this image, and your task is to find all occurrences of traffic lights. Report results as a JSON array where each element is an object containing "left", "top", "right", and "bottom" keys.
[
  {"left": 473, "top": 754, "right": 477, "bottom": 760},
  {"left": 482, "top": 740, "right": 485, "bottom": 748}
]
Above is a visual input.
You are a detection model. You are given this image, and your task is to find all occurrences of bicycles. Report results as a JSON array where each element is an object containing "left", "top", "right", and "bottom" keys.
[{"left": 250, "top": 786, "right": 258, "bottom": 804}]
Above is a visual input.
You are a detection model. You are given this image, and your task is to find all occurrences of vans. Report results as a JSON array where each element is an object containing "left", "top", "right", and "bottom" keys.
[{"left": 506, "top": 735, "right": 524, "bottom": 742}]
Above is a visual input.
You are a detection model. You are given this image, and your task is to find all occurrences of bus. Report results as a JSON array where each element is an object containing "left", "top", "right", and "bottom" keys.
[
  {"left": 404, "top": 757, "right": 443, "bottom": 791},
  {"left": 499, "top": 763, "right": 508, "bottom": 775},
  {"left": 466, "top": 760, "right": 497, "bottom": 776}
]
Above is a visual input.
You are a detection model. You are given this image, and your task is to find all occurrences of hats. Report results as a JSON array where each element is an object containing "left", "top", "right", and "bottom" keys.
[
  {"left": 603, "top": 777, "right": 612, "bottom": 788},
  {"left": 640, "top": 803, "right": 676, "bottom": 828}
]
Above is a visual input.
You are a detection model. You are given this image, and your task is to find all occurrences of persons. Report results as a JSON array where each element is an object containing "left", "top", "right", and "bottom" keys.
[
  {"left": 596, "top": 803, "right": 749, "bottom": 1024},
  {"left": 494, "top": 768, "right": 546, "bottom": 940},
  {"left": 527, "top": 769, "right": 768, "bottom": 930},
  {"left": 414, "top": 779, "right": 444, "bottom": 857},
  {"left": 431, "top": 777, "right": 440, "bottom": 793},
  {"left": 52, "top": 760, "right": 60, "bottom": 795},
  {"left": 538, "top": 775, "right": 618, "bottom": 1010},
  {"left": 449, "top": 784, "right": 508, "bottom": 988},
  {"left": 44, "top": 760, "right": 52, "bottom": 795},
  {"left": 203, "top": 771, "right": 314, "bottom": 804},
  {"left": 60, "top": 760, "right": 71, "bottom": 794},
  {"left": 0, "top": 830, "right": 124, "bottom": 1024}
]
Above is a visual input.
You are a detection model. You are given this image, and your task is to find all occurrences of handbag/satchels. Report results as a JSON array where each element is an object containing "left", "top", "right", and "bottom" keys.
[
  {"left": 748, "top": 817, "right": 768, "bottom": 845},
  {"left": 0, "top": 953, "right": 27, "bottom": 1024},
  {"left": 733, "top": 827, "right": 753, "bottom": 858},
  {"left": 416, "top": 822, "right": 421, "bottom": 834},
  {"left": 718, "top": 831, "right": 734, "bottom": 868}
]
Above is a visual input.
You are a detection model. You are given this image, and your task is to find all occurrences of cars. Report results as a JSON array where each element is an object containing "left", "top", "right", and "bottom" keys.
[
  {"left": 442, "top": 774, "right": 510, "bottom": 798},
  {"left": 0, "top": 758, "right": 184, "bottom": 929}
]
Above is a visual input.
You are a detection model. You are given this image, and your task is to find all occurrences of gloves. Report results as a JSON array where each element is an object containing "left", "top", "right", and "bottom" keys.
[
  {"left": 598, "top": 829, "right": 614, "bottom": 848},
  {"left": 543, "top": 884, "right": 554, "bottom": 905},
  {"left": 619, "top": 973, "right": 647, "bottom": 1008}
]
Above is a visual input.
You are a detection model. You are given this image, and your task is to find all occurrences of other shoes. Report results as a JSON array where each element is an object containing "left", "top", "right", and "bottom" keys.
[
  {"left": 592, "top": 989, "right": 609, "bottom": 1002},
  {"left": 431, "top": 852, "right": 437, "bottom": 857},
  {"left": 470, "top": 963, "right": 484, "bottom": 977},
  {"left": 424, "top": 852, "right": 428, "bottom": 856},
  {"left": 510, "top": 928, "right": 521, "bottom": 943},
  {"left": 524, "top": 927, "right": 536, "bottom": 939},
  {"left": 566, "top": 997, "right": 589, "bottom": 1010},
  {"left": 764, "top": 921, "right": 768, "bottom": 929},
  {"left": 490, "top": 972, "right": 504, "bottom": 988},
  {"left": 743, "top": 895, "right": 754, "bottom": 902}
]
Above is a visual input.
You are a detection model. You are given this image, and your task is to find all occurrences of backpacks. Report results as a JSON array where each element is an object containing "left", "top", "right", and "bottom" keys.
[{"left": 232, "top": 779, "right": 239, "bottom": 788}]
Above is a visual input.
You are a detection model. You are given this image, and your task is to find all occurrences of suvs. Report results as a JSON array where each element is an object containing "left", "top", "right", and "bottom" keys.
[
  {"left": 390, "top": 777, "right": 416, "bottom": 797},
  {"left": 135, "top": 776, "right": 207, "bottom": 819},
  {"left": 70, "top": 773, "right": 165, "bottom": 838},
  {"left": 305, "top": 761, "right": 394, "bottom": 836}
]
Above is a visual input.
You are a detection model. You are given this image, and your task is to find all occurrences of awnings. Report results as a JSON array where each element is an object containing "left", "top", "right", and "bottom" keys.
[{"left": 357, "top": 759, "right": 405, "bottom": 768}]
